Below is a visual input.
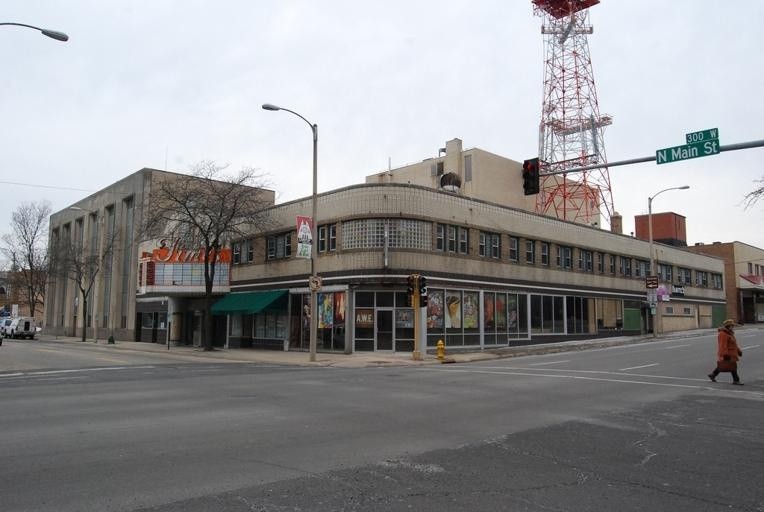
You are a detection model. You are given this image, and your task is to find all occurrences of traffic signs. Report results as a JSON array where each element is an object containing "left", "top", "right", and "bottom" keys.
[
  {"left": 655, "top": 139, "right": 720, "bottom": 164},
  {"left": 646, "top": 276, "right": 658, "bottom": 288}
]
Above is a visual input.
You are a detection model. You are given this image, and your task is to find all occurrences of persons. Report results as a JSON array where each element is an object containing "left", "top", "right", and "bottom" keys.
[{"left": 706, "top": 320, "right": 745, "bottom": 385}]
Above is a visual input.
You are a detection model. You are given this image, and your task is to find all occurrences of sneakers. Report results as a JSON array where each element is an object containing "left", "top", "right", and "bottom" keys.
[
  {"left": 708, "top": 374, "right": 716, "bottom": 382},
  {"left": 733, "top": 381, "right": 743, "bottom": 385}
]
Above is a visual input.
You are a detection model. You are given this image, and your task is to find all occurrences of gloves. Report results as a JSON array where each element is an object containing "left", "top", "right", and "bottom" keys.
[
  {"left": 724, "top": 355, "right": 730, "bottom": 361},
  {"left": 738, "top": 351, "right": 742, "bottom": 356}
]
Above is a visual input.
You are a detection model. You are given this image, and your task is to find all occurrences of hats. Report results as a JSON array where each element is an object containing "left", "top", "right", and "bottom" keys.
[{"left": 723, "top": 320, "right": 736, "bottom": 326}]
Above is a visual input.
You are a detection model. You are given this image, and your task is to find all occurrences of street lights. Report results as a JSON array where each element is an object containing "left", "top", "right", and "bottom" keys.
[
  {"left": 261, "top": 103, "right": 318, "bottom": 361},
  {"left": 69, "top": 207, "right": 104, "bottom": 342},
  {"left": 0, "top": 22, "right": 68, "bottom": 42},
  {"left": 648, "top": 186, "right": 690, "bottom": 338}
]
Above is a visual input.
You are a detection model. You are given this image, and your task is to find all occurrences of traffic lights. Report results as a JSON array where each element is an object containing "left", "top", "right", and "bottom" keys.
[
  {"left": 522, "top": 158, "right": 539, "bottom": 195},
  {"left": 419, "top": 277, "right": 427, "bottom": 293},
  {"left": 407, "top": 276, "right": 414, "bottom": 295}
]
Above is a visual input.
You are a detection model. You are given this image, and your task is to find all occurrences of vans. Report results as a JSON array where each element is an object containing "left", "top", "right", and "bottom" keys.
[{"left": 331, "top": 324, "right": 372, "bottom": 349}]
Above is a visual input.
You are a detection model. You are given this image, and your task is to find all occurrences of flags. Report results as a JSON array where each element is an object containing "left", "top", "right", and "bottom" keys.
[{"left": 294, "top": 216, "right": 313, "bottom": 259}]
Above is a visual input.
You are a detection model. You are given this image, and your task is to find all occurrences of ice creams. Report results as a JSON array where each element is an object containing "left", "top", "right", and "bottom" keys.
[{"left": 446, "top": 296, "right": 459, "bottom": 318}]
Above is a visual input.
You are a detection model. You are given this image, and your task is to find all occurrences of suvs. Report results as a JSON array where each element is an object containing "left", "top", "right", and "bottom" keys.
[{"left": 0, "top": 317, "right": 37, "bottom": 344}]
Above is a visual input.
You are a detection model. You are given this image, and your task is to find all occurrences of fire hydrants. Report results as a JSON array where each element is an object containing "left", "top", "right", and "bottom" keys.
[{"left": 437, "top": 340, "right": 445, "bottom": 360}]
